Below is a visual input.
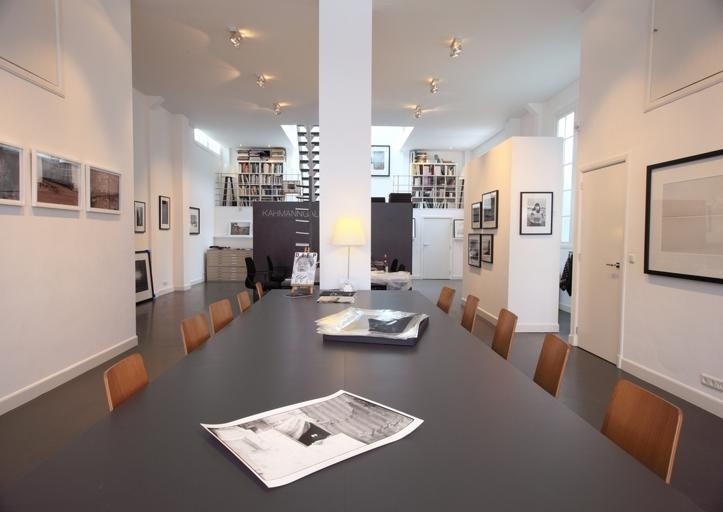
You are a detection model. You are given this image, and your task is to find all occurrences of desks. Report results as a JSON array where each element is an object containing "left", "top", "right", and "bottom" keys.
[{"left": 1, "top": 292, "right": 702, "bottom": 512}]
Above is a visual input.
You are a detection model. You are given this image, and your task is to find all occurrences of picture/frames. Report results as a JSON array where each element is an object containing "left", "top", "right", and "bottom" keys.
[
  {"left": 412, "top": 218, "right": 417, "bottom": 241},
  {"left": 1, "top": 142, "right": 123, "bottom": 214},
  {"left": 452, "top": 190, "right": 499, "bottom": 267},
  {"left": 290, "top": 252, "right": 317, "bottom": 286},
  {"left": 134, "top": 195, "right": 200, "bottom": 304},
  {"left": 646, "top": 149, "right": 723, "bottom": 285},
  {"left": 371, "top": 145, "right": 390, "bottom": 177},
  {"left": 229, "top": 220, "right": 253, "bottom": 237},
  {"left": 409, "top": 150, "right": 464, "bottom": 208},
  {"left": 519, "top": 191, "right": 553, "bottom": 235}
]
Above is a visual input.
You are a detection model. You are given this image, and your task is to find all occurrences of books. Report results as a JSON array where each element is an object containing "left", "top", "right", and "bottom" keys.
[
  {"left": 411, "top": 150, "right": 458, "bottom": 209},
  {"left": 237, "top": 147, "right": 287, "bottom": 206}
]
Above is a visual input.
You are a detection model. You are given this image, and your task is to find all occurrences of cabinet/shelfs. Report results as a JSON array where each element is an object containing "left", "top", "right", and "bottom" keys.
[{"left": 229, "top": 146, "right": 287, "bottom": 206}]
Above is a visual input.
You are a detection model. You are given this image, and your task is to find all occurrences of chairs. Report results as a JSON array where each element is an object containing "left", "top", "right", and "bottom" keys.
[
  {"left": 460, "top": 294, "right": 480, "bottom": 333},
  {"left": 371, "top": 247, "right": 405, "bottom": 273},
  {"left": 491, "top": 308, "right": 519, "bottom": 360},
  {"left": 600, "top": 379, "right": 684, "bottom": 486},
  {"left": 104, "top": 353, "right": 149, "bottom": 413},
  {"left": 209, "top": 299, "right": 234, "bottom": 334},
  {"left": 436, "top": 286, "right": 456, "bottom": 314},
  {"left": 244, "top": 255, "right": 287, "bottom": 299},
  {"left": 532, "top": 332, "right": 570, "bottom": 397},
  {"left": 181, "top": 313, "right": 211, "bottom": 355},
  {"left": 234, "top": 289, "right": 253, "bottom": 312}
]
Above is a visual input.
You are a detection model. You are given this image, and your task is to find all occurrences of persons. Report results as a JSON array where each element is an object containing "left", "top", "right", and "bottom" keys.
[
  {"left": 530, "top": 203, "right": 544, "bottom": 225},
  {"left": 295, "top": 255, "right": 314, "bottom": 284}
]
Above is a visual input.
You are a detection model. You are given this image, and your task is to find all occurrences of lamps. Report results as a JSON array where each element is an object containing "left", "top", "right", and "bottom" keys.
[
  {"left": 414, "top": 38, "right": 462, "bottom": 121},
  {"left": 331, "top": 213, "right": 366, "bottom": 294},
  {"left": 225, "top": 28, "right": 281, "bottom": 125}
]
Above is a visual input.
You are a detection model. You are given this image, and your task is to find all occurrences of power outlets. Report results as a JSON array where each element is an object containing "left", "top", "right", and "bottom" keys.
[{"left": 700, "top": 373, "right": 723, "bottom": 393}]
